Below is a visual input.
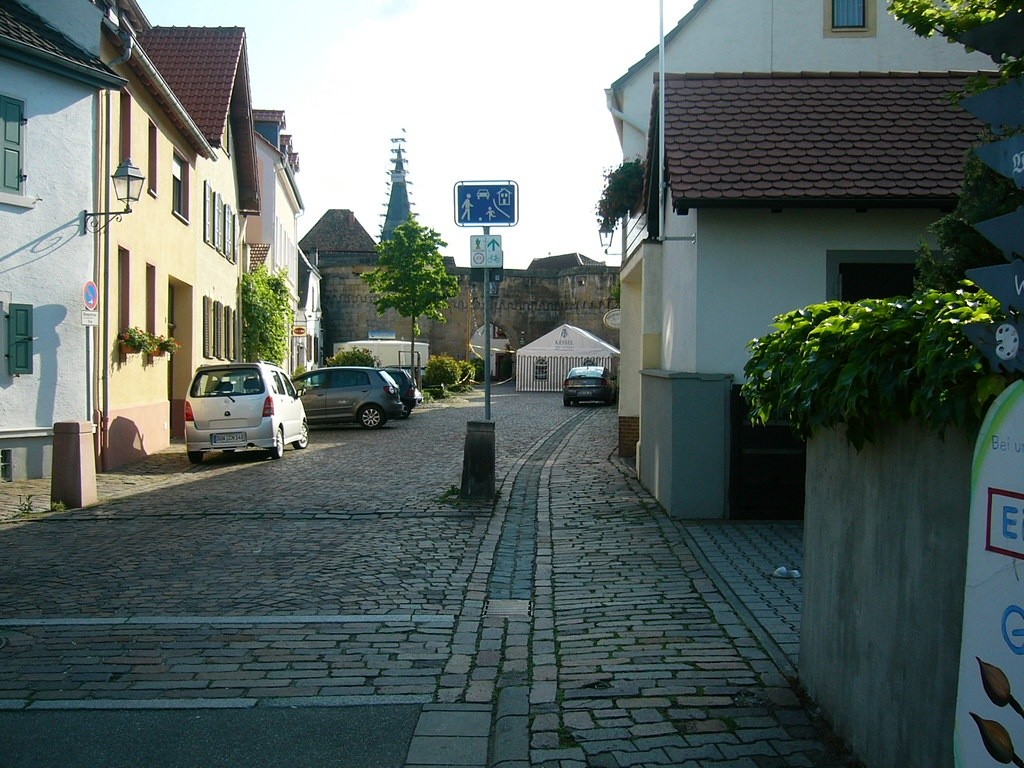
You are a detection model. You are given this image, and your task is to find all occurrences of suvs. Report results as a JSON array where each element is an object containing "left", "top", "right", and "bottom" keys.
[
  {"left": 292, "top": 366, "right": 406, "bottom": 429},
  {"left": 381, "top": 368, "right": 423, "bottom": 421}
]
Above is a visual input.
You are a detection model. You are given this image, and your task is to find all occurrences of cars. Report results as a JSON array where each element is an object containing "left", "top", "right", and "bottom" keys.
[
  {"left": 563, "top": 366, "right": 617, "bottom": 406},
  {"left": 183, "top": 360, "right": 310, "bottom": 464}
]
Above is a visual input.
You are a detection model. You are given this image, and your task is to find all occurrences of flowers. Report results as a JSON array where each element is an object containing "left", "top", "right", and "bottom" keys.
[
  {"left": 596, "top": 154, "right": 651, "bottom": 230},
  {"left": 118, "top": 327, "right": 150, "bottom": 350},
  {"left": 142, "top": 336, "right": 183, "bottom": 355}
]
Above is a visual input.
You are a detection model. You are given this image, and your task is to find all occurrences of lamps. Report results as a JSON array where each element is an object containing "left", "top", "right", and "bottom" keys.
[
  {"left": 598, "top": 219, "right": 622, "bottom": 256},
  {"left": 83, "top": 155, "right": 146, "bottom": 235}
]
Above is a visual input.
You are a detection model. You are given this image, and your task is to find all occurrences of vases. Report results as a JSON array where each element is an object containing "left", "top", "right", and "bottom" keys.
[
  {"left": 120, "top": 343, "right": 140, "bottom": 354},
  {"left": 147, "top": 345, "right": 166, "bottom": 357}
]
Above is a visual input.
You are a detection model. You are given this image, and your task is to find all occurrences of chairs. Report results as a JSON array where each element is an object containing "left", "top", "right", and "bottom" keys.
[
  {"left": 214, "top": 381, "right": 234, "bottom": 393},
  {"left": 243, "top": 377, "right": 260, "bottom": 393}
]
